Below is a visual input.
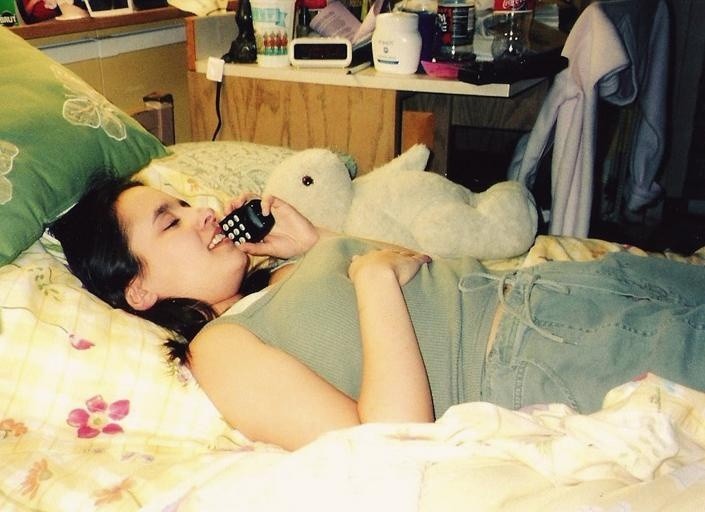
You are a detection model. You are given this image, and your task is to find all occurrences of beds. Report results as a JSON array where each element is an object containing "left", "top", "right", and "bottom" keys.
[{"left": 0, "top": 240, "right": 705, "bottom": 510}]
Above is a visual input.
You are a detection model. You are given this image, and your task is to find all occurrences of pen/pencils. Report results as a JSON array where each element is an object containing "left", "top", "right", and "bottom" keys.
[{"left": 347, "top": 61, "right": 371, "bottom": 74}]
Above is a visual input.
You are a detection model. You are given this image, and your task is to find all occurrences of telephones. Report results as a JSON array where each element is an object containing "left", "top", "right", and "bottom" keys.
[{"left": 219, "top": 200, "right": 274, "bottom": 244}]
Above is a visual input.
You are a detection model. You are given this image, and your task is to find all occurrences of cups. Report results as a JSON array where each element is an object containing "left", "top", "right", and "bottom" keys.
[{"left": 403, "top": 10, "right": 436, "bottom": 71}]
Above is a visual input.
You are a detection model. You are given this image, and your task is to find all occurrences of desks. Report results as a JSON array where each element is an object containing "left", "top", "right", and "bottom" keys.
[{"left": 188, "top": 61, "right": 547, "bottom": 178}]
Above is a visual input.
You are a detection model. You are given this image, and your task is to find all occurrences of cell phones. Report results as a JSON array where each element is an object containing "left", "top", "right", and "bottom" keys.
[{"left": 215, "top": 198, "right": 275, "bottom": 249}]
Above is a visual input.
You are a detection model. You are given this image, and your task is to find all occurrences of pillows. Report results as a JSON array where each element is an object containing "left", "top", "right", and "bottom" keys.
[
  {"left": 35, "top": 141, "right": 360, "bottom": 296},
  {"left": 0, "top": 23, "right": 173, "bottom": 268}
]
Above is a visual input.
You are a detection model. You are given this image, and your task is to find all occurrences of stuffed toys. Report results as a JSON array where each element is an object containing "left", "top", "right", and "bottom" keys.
[{"left": 262, "top": 143, "right": 540, "bottom": 263}]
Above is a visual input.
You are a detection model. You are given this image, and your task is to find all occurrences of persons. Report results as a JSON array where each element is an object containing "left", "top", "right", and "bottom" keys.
[{"left": 47, "top": 165, "right": 705, "bottom": 455}]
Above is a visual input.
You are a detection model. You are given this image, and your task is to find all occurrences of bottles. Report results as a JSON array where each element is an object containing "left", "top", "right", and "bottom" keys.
[
  {"left": 437, "top": 0, "right": 535, "bottom": 61},
  {"left": 296, "top": 7, "right": 311, "bottom": 39}
]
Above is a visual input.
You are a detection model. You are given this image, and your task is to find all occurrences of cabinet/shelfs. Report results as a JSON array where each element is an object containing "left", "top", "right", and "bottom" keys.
[{"left": 10, "top": 6, "right": 203, "bottom": 144}]
